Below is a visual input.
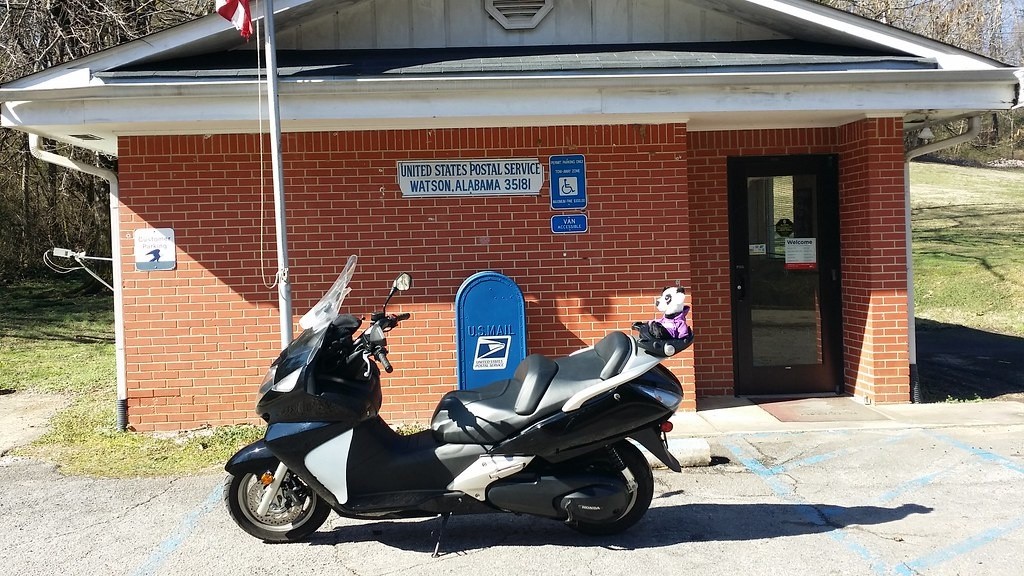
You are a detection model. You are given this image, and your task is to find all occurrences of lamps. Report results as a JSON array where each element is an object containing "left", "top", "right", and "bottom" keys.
[{"left": 918, "top": 128, "right": 935, "bottom": 140}]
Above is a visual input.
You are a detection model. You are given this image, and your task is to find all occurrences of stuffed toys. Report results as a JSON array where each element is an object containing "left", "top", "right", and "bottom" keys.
[{"left": 648, "top": 286, "right": 690, "bottom": 340}]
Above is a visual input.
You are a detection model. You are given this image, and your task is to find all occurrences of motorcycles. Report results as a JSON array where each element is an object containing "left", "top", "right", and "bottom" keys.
[{"left": 219, "top": 254, "right": 701, "bottom": 560}]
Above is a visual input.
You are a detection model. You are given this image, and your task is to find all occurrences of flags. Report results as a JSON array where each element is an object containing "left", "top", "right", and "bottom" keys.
[{"left": 215, "top": 0, "right": 253, "bottom": 43}]
